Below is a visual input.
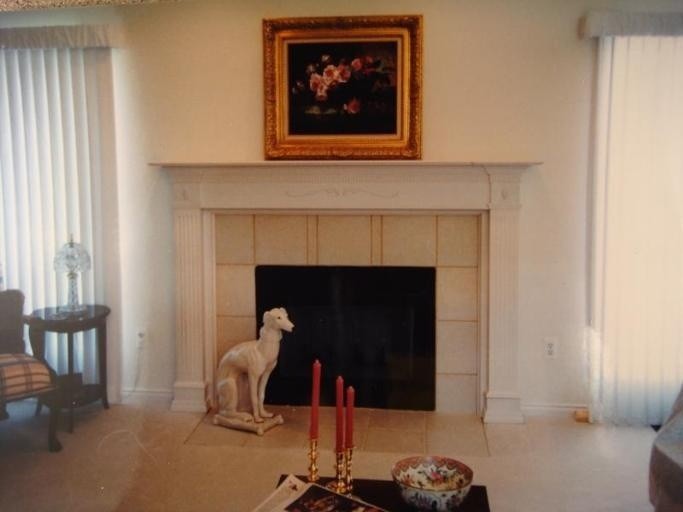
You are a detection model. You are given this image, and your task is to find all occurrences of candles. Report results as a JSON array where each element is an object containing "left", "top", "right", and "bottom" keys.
[
  {"left": 344, "top": 386, "right": 353, "bottom": 446},
  {"left": 336, "top": 376, "right": 343, "bottom": 448},
  {"left": 309, "top": 360, "right": 319, "bottom": 436}
]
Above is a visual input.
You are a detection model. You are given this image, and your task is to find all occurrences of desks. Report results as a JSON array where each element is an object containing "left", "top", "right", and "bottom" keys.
[
  {"left": 21, "top": 304, "right": 109, "bottom": 416},
  {"left": 276, "top": 471, "right": 489, "bottom": 512}
]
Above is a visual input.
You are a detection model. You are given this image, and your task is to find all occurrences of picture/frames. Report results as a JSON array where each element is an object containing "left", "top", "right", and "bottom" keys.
[{"left": 260, "top": 14, "right": 421, "bottom": 159}]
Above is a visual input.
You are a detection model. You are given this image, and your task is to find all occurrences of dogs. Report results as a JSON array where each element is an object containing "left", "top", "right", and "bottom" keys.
[{"left": 215, "top": 308, "right": 297, "bottom": 424}]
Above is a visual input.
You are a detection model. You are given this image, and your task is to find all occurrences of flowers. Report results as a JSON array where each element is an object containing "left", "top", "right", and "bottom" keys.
[{"left": 289, "top": 52, "right": 388, "bottom": 115}]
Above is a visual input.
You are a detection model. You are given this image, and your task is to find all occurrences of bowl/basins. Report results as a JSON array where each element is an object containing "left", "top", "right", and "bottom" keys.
[{"left": 390, "top": 456, "right": 473, "bottom": 512}]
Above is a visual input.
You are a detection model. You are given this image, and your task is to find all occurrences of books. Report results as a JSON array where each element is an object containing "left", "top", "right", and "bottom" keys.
[{"left": 252, "top": 475, "right": 389, "bottom": 511}]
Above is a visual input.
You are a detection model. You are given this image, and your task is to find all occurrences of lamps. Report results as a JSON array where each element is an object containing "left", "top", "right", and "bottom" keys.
[{"left": 51, "top": 235, "right": 91, "bottom": 315}]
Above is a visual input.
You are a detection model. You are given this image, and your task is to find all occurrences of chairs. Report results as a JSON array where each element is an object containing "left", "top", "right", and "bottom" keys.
[{"left": 0, "top": 289, "right": 61, "bottom": 456}]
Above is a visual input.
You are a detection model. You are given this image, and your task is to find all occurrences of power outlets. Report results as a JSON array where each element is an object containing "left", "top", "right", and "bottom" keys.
[{"left": 542, "top": 338, "right": 557, "bottom": 357}]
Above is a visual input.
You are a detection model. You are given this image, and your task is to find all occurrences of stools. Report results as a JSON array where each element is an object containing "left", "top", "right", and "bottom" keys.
[{"left": 648, "top": 381, "right": 682, "bottom": 512}]
